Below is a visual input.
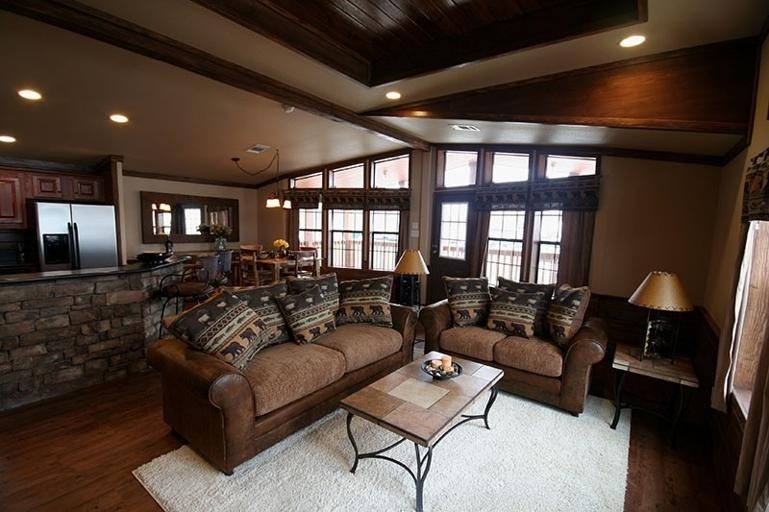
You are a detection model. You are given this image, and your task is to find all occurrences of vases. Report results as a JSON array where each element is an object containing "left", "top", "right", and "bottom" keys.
[{"left": 212, "top": 237, "right": 226, "bottom": 254}]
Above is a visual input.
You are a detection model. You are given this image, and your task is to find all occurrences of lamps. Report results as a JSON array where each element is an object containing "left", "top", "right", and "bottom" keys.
[
  {"left": 263, "top": 149, "right": 294, "bottom": 210},
  {"left": 626, "top": 270, "right": 694, "bottom": 363},
  {"left": 394, "top": 250, "right": 429, "bottom": 308}
]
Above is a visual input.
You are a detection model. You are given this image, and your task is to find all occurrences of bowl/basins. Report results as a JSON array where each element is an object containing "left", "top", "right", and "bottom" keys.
[
  {"left": 136, "top": 252, "right": 172, "bottom": 263},
  {"left": 420, "top": 359, "right": 463, "bottom": 380},
  {"left": 256, "top": 254, "right": 269, "bottom": 259}
]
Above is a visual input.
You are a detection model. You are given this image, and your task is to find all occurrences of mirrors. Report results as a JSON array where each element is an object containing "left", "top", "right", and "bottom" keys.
[{"left": 139, "top": 190, "right": 244, "bottom": 244}]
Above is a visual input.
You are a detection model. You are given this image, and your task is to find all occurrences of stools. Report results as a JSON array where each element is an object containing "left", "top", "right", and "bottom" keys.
[{"left": 159, "top": 264, "right": 215, "bottom": 338}]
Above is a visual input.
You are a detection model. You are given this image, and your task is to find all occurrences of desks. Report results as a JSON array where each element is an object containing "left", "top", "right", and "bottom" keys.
[{"left": 610, "top": 340, "right": 701, "bottom": 441}]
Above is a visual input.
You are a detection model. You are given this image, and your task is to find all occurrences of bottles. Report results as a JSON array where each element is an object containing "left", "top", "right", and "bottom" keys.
[{"left": 165, "top": 237, "right": 173, "bottom": 254}]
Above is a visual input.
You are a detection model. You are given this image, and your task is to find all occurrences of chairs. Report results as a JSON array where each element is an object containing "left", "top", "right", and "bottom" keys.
[{"left": 232, "top": 242, "right": 323, "bottom": 286}]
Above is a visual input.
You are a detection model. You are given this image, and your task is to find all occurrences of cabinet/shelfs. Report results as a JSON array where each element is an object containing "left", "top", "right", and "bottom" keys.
[
  {"left": 28, "top": 171, "right": 104, "bottom": 203},
  {"left": 0, "top": 170, "right": 28, "bottom": 230}
]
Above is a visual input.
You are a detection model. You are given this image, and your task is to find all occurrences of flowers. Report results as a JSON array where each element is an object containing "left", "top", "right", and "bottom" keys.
[
  {"left": 197, "top": 220, "right": 210, "bottom": 235},
  {"left": 273, "top": 238, "right": 290, "bottom": 249},
  {"left": 204, "top": 221, "right": 232, "bottom": 237}
]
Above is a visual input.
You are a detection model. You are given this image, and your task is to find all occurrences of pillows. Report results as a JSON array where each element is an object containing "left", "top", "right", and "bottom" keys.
[
  {"left": 441, "top": 274, "right": 591, "bottom": 348},
  {"left": 158, "top": 272, "right": 395, "bottom": 372}
]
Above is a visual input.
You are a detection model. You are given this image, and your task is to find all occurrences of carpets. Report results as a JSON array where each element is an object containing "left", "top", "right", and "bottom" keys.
[{"left": 131, "top": 380, "right": 633, "bottom": 511}]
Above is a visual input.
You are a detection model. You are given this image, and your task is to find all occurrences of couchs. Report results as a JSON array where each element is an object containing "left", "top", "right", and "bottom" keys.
[
  {"left": 148, "top": 269, "right": 419, "bottom": 477},
  {"left": 419, "top": 286, "right": 608, "bottom": 418}
]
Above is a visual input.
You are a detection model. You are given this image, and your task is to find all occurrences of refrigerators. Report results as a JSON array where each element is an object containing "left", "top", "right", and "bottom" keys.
[{"left": 29, "top": 200, "right": 119, "bottom": 271}]
